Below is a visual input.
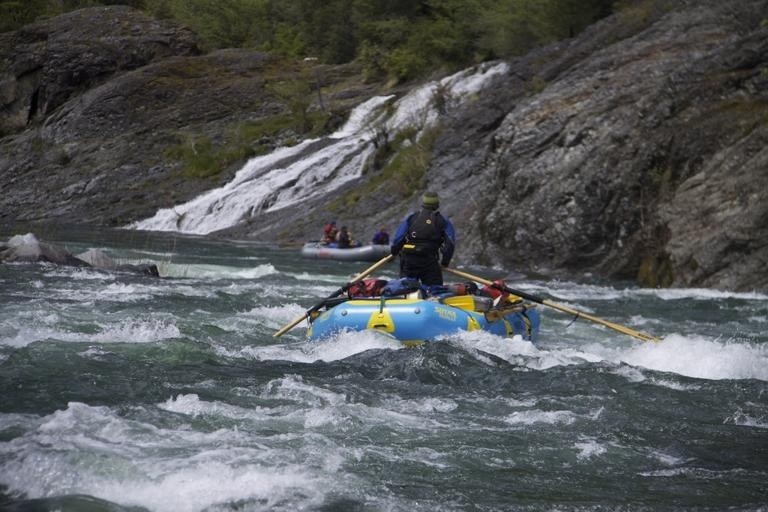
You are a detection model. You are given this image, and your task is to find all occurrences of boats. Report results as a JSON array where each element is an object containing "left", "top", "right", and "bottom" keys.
[
  {"left": 299, "top": 239, "right": 395, "bottom": 264},
  {"left": 301, "top": 288, "right": 545, "bottom": 348}
]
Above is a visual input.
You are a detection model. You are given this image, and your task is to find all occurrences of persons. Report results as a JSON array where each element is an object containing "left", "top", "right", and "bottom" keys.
[
  {"left": 373, "top": 226, "right": 389, "bottom": 245},
  {"left": 335, "top": 226, "right": 352, "bottom": 248},
  {"left": 321, "top": 220, "right": 338, "bottom": 244},
  {"left": 390, "top": 190, "right": 456, "bottom": 286}
]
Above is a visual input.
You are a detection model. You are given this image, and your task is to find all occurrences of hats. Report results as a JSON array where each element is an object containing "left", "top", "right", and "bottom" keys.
[{"left": 423, "top": 192, "right": 439, "bottom": 210}]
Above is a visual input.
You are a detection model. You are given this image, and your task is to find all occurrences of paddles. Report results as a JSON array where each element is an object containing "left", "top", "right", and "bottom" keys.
[
  {"left": 438, "top": 264, "right": 660, "bottom": 342},
  {"left": 444, "top": 295, "right": 475, "bottom": 314}
]
